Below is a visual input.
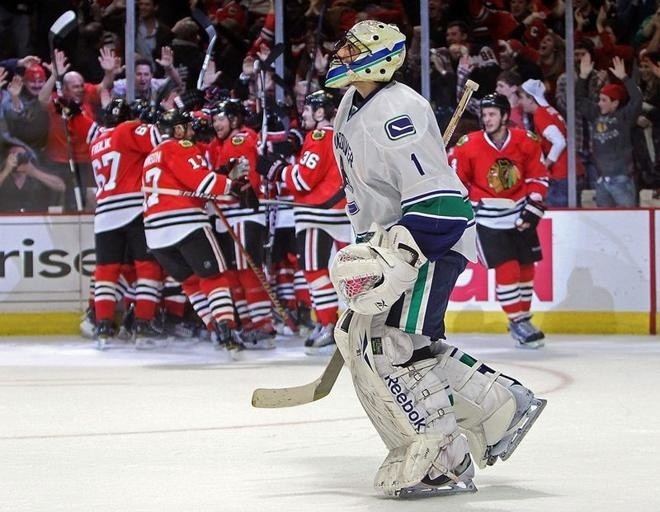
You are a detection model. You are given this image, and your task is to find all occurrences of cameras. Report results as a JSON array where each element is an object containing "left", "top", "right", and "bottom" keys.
[{"left": 17, "top": 152, "right": 31, "bottom": 164}]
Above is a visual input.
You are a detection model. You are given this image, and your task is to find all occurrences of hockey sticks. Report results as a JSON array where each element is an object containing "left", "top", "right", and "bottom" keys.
[
  {"left": 48, "top": 10, "right": 84, "bottom": 212},
  {"left": 141, "top": 186, "right": 346, "bottom": 209},
  {"left": 194, "top": 10, "right": 217, "bottom": 88},
  {"left": 252, "top": 78, "right": 479, "bottom": 413},
  {"left": 211, "top": 199, "right": 299, "bottom": 334},
  {"left": 261, "top": 43, "right": 296, "bottom": 272}
]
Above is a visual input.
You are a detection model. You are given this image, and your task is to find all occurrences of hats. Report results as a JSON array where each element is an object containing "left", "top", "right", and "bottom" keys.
[
  {"left": 24, "top": 64, "right": 46, "bottom": 81},
  {"left": 170, "top": 17, "right": 200, "bottom": 37},
  {"left": 521, "top": 79, "right": 550, "bottom": 107},
  {"left": 600, "top": 84, "right": 627, "bottom": 100}
]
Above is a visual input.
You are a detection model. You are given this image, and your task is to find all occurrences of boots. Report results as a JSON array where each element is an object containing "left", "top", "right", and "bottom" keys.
[{"left": 87, "top": 303, "right": 336, "bottom": 350}]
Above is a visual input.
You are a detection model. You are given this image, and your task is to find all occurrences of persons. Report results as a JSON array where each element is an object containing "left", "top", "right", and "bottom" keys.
[{"left": 323, "top": 19, "right": 535, "bottom": 499}]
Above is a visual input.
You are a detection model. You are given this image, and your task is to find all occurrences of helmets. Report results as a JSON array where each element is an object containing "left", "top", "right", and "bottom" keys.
[
  {"left": 325, "top": 20, "right": 406, "bottom": 89},
  {"left": 481, "top": 93, "right": 510, "bottom": 110},
  {"left": 105, "top": 91, "right": 336, "bottom": 139}
]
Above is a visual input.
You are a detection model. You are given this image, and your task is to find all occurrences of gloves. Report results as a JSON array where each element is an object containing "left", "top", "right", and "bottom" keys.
[
  {"left": 256, "top": 145, "right": 291, "bottom": 182},
  {"left": 498, "top": 40, "right": 516, "bottom": 58},
  {"left": 516, "top": 196, "right": 547, "bottom": 236}
]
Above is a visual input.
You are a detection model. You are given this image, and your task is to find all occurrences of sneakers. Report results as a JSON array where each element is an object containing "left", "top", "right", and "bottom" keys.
[
  {"left": 509, "top": 315, "right": 544, "bottom": 343},
  {"left": 400, "top": 451, "right": 473, "bottom": 489},
  {"left": 488, "top": 386, "right": 533, "bottom": 456}
]
